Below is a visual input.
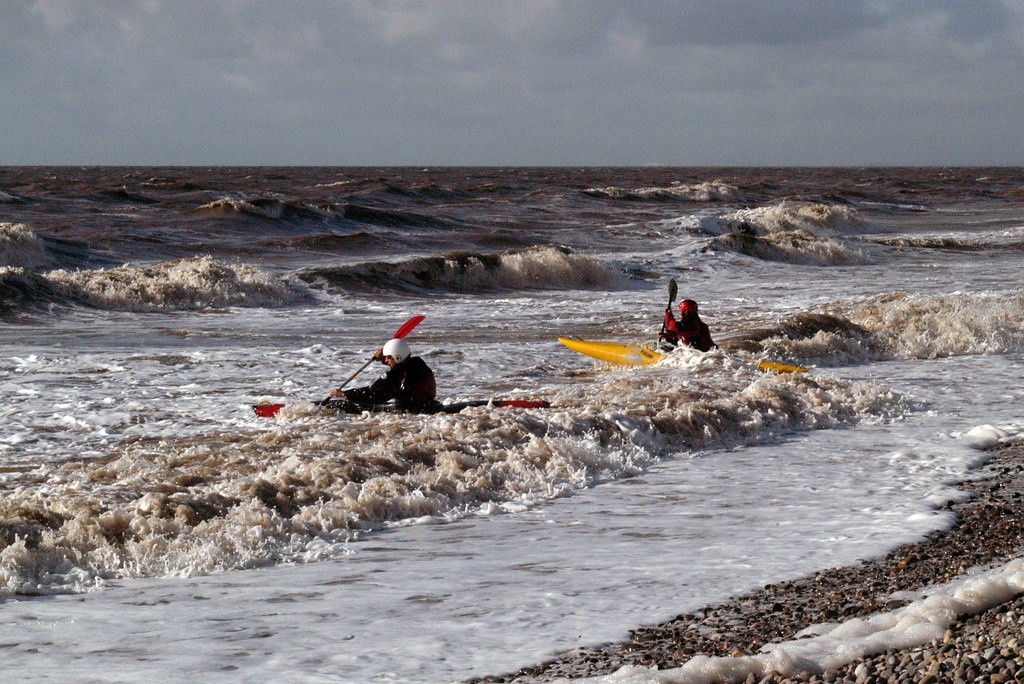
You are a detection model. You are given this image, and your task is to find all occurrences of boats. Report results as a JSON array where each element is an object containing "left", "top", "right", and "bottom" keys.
[
  {"left": 252, "top": 400, "right": 550, "bottom": 415},
  {"left": 557, "top": 337, "right": 809, "bottom": 374}
]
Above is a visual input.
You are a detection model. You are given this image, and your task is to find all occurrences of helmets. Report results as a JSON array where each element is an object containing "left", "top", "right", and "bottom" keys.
[
  {"left": 382, "top": 338, "right": 411, "bottom": 363},
  {"left": 679, "top": 299, "right": 699, "bottom": 314}
]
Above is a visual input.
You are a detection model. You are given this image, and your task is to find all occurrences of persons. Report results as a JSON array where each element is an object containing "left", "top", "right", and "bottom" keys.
[
  {"left": 326, "top": 338, "right": 436, "bottom": 414},
  {"left": 662, "top": 300, "right": 718, "bottom": 352}
]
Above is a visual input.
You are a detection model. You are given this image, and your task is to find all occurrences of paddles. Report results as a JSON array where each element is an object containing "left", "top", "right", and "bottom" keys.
[
  {"left": 658, "top": 278, "right": 678, "bottom": 343},
  {"left": 320, "top": 315, "right": 427, "bottom": 407}
]
